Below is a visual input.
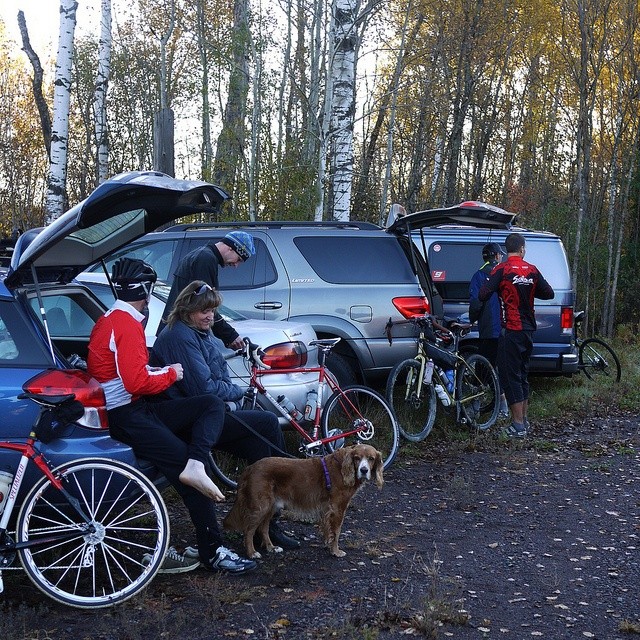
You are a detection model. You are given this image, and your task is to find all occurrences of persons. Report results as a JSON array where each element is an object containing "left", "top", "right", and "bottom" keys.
[
  {"left": 148, "top": 279, "right": 304, "bottom": 551},
  {"left": 478, "top": 232, "right": 556, "bottom": 440},
  {"left": 154, "top": 231, "right": 257, "bottom": 351},
  {"left": 85, "top": 254, "right": 258, "bottom": 576},
  {"left": 464, "top": 242, "right": 512, "bottom": 422}
]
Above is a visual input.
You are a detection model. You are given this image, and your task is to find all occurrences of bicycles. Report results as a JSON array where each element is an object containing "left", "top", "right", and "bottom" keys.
[
  {"left": 208, "top": 337, "right": 400, "bottom": 492},
  {"left": 384, "top": 313, "right": 499, "bottom": 443},
  {"left": 573, "top": 311, "right": 620, "bottom": 385},
  {"left": 0, "top": 392, "right": 172, "bottom": 610}
]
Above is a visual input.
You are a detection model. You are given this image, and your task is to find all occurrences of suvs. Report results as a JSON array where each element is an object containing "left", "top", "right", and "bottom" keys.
[
  {"left": 0, "top": 170, "right": 231, "bottom": 562},
  {"left": 88, "top": 198, "right": 518, "bottom": 408}
]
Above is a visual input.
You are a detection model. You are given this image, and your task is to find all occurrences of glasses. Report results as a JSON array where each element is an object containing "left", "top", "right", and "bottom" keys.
[
  {"left": 193, "top": 282, "right": 212, "bottom": 295},
  {"left": 501, "top": 254, "right": 503, "bottom": 257}
]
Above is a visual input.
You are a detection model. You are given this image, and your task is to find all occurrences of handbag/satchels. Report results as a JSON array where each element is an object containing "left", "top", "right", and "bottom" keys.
[{"left": 423, "top": 341, "right": 458, "bottom": 370}]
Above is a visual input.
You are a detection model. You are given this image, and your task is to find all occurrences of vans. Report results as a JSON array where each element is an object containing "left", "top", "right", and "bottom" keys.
[{"left": 385, "top": 228, "right": 581, "bottom": 379}]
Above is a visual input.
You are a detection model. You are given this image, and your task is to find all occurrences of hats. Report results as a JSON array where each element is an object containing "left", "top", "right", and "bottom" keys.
[
  {"left": 223, "top": 230, "right": 256, "bottom": 262},
  {"left": 482, "top": 243, "right": 507, "bottom": 256}
]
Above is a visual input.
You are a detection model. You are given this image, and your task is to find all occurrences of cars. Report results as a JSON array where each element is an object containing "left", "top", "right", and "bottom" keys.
[{"left": 71, "top": 271, "right": 342, "bottom": 426}]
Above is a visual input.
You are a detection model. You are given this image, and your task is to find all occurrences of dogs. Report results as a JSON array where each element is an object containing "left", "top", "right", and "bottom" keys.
[{"left": 221, "top": 443, "right": 386, "bottom": 562}]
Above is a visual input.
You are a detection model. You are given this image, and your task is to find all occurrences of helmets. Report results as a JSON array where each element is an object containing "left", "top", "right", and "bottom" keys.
[{"left": 111, "top": 258, "right": 157, "bottom": 300}]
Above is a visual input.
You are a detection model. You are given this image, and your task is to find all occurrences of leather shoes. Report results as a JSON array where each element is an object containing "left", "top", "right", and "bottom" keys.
[{"left": 269, "top": 519, "right": 301, "bottom": 547}]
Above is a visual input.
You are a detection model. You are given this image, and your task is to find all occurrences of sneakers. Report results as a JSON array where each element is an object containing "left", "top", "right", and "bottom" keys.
[
  {"left": 67, "top": 353, "right": 87, "bottom": 369},
  {"left": 470, "top": 410, "right": 480, "bottom": 419},
  {"left": 180, "top": 546, "right": 205, "bottom": 567},
  {"left": 496, "top": 409, "right": 511, "bottom": 420},
  {"left": 140, "top": 546, "right": 200, "bottom": 574},
  {"left": 204, "top": 545, "right": 257, "bottom": 575},
  {"left": 495, "top": 422, "right": 527, "bottom": 438},
  {"left": 524, "top": 420, "right": 530, "bottom": 431}
]
